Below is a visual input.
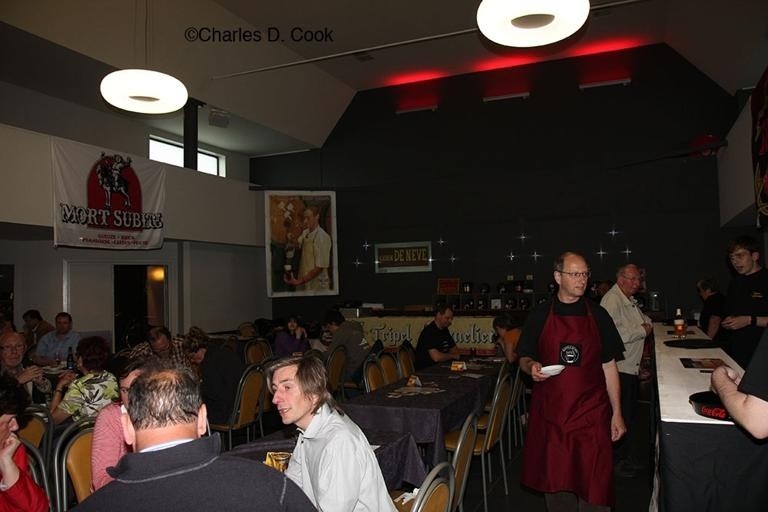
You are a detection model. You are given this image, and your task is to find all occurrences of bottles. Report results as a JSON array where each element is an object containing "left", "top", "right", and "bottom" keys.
[
  {"left": 67, "top": 346, "right": 74, "bottom": 370},
  {"left": 676, "top": 308, "right": 682, "bottom": 319}
]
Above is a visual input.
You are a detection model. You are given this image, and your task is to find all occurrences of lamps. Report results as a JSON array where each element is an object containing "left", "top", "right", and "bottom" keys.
[
  {"left": 476, "top": 0, "right": 591, "bottom": 48},
  {"left": 100, "top": 0, "right": 189, "bottom": 114}
]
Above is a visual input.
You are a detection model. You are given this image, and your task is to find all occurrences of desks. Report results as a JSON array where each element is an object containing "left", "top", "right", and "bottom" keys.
[{"left": 652, "top": 322, "right": 746, "bottom": 512}]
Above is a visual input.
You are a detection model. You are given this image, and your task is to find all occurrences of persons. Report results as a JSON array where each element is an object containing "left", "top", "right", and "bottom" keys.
[
  {"left": 698, "top": 280, "right": 724, "bottom": 338},
  {"left": 722, "top": 236, "right": 766, "bottom": 364},
  {"left": 517, "top": 251, "right": 629, "bottom": 511},
  {"left": 129, "top": 327, "right": 251, "bottom": 424},
  {"left": 416, "top": 304, "right": 460, "bottom": 367},
  {"left": 286, "top": 205, "right": 332, "bottom": 289},
  {"left": 493, "top": 313, "right": 523, "bottom": 365},
  {"left": 265, "top": 355, "right": 398, "bottom": 512},
  {"left": 1, "top": 370, "right": 49, "bottom": 512},
  {"left": 1, "top": 311, "right": 120, "bottom": 428},
  {"left": 70, "top": 363, "right": 318, "bottom": 512},
  {"left": 750, "top": 314, "right": 757, "bottom": 328},
  {"left": 274, "top": 310, "right": 369, "bottom": 382},
  {"left": 711, "top": 341, "right": 768, "bottom": 455},
  {"left": 600, "top": 265, "right": 653, "bottom": 478}
]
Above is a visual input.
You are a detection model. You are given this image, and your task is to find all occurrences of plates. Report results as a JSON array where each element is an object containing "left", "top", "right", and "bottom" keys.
[{"left": 539, "top": 364, "right": 565, "bottom": 376}]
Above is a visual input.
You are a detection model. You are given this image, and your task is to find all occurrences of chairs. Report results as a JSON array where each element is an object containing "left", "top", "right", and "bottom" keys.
[{"left": 18, "top": 332, "right": 132, "bottom": 512}]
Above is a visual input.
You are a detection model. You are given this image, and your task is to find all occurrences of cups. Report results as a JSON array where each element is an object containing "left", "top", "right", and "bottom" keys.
[
  {"left": 674, "top": 319, "right": 685, "bottom": 336},
  {"left": 54, "top": 351, "right": 62, "bottom": 364}
]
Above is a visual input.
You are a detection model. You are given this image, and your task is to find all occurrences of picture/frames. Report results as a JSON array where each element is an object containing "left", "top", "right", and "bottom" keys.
[
  {"left": 373, "top": 238, "right": 434, "bottom": 275},
  {"left": 264, "top": 189, "right": 340, "bottom": 299}
]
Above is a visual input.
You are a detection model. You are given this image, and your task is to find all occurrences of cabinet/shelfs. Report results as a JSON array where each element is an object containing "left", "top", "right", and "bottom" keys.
[{"left": 433, "top": 273, "right": 551, "bottom": 310}]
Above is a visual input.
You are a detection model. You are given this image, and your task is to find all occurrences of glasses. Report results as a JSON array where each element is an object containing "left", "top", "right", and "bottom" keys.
[
  {"left": 559, "top": 271, "right": 591, "bottom": 277},
  {"left": 624, "top": 277, "right": 640, "bottom": 281}
]
